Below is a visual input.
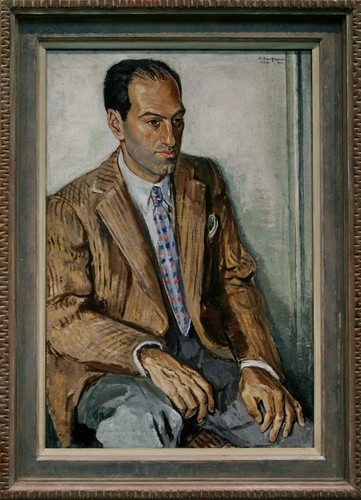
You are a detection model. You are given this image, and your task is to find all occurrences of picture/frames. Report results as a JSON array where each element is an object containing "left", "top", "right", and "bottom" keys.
[{"left": 0, "top": 0, "right": 360, "bottom": 500}]
